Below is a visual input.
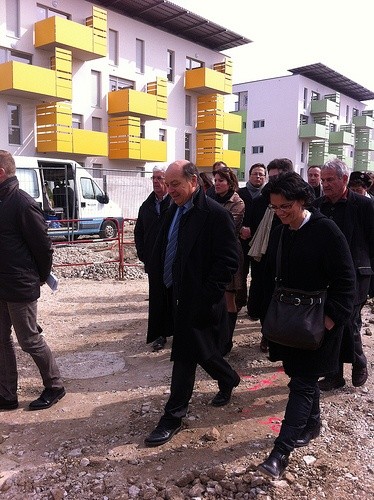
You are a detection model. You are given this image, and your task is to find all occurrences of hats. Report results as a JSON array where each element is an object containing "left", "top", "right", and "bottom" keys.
[{"left": 350, "top": 171, "right": 372, "bottom": 190}]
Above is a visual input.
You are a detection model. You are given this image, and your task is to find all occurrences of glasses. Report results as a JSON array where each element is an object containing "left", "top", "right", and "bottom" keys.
[
  {"left": 250, "top": 172, "right": 266, "bottom": 178},
  {"left": 265, "top": 198, "right": 296, "bottom": 212},
  {"left": 150, "top": 176, "right": 166, "bottom": 180}
]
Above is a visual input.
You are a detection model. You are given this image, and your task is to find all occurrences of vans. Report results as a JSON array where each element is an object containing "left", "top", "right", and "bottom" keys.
[{"left": 10, "top": 155, "right": 123, "bottom": 240}]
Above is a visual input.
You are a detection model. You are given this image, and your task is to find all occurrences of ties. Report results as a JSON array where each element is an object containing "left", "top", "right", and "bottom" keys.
[{"left": 163, "top": 205, "right": 187, "bottom": 288}]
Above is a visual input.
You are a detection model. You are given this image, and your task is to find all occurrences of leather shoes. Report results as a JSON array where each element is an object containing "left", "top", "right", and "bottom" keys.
[
  {"left": 145, "top": 415, "right": 184, "bottom": 446},
  {"left": 211, "top": 371, "right": 240, "bottom": 406},
  {"left": 29, "top": 387, "right": 66, "bottom": 410},
  {"left": 0, "top": 397, "right": 19, "bottom": 410}
]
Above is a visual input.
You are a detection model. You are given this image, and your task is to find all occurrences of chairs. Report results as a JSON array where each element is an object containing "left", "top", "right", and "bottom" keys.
[{"left": 42, "top": 178, "right": 79, "bottom": 217}]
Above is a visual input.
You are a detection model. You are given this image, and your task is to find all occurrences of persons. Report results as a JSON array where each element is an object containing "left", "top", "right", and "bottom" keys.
[
  {"left": 247, "top": 157, "right": 295, "bottom": 353},
  {"left": 238, "top": 163, "right": 267, "bottom": 321},
  {"left": 256, "top": 173, "right": 357, "bottom": 480},
  {"left": 312, "top": 158, "right": 374, "bottom": 392},
  {"left": 0, "top": 149, "right": 69, "bottom": 412},
  {"left": 142, "top": 159, "right": 245, "bottom": 448},
  {"left": 132, "top": 165, "right": 175, "bottom": 350},
  {"left": 307, "top": 165, "right": 328, "bottom": 202}
]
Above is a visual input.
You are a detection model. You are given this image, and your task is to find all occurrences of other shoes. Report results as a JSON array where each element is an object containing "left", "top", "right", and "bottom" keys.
[{"left": 153, "top": 335, "right": 167, "bottom": 351}]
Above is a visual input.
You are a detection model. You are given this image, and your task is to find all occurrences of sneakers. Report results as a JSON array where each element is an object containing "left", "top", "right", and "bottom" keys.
[
  {"left": 317, "top": 375, "right": 346, "bottom": 392},
  {"left": 352, "top": 360, "right": 369, "bottom": 387}
]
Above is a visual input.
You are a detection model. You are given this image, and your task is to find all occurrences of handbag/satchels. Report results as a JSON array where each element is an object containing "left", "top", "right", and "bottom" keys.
[{"left": 261, "top": 286, "right": 327, "bottom": 350}]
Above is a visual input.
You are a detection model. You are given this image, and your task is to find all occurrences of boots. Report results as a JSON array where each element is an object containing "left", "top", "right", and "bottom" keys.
[
  {"left": 295, "top": 415, "right": 322, "bottom": 446},
  {"left": 258, "top": 437, "right": 293, "bottom": 478}
]
